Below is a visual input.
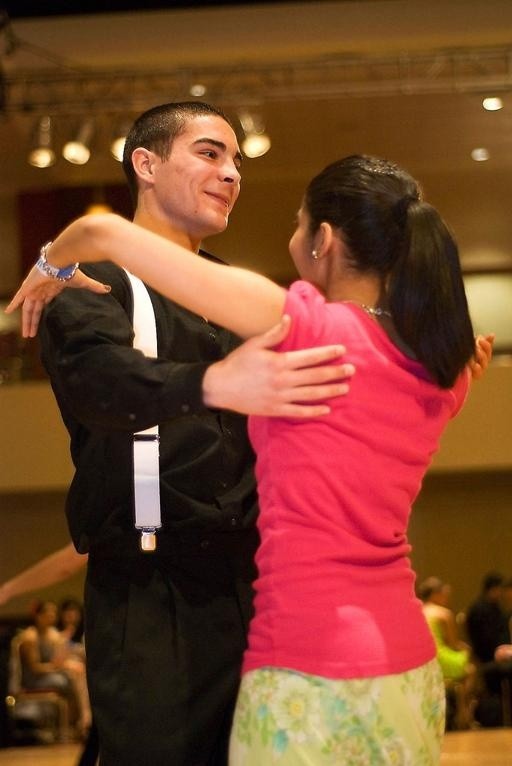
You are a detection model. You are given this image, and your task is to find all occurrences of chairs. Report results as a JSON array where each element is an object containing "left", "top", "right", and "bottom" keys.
[{"left": 8, "top": 633, "right": 70, "bottom": 743}]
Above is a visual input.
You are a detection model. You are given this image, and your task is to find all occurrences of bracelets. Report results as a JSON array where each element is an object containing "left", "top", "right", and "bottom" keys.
[
  {"left": 36, "top": 241, "right": 80, "bottom": 283},
  {"left": 360, "top": 303, "right": 396, "bottom": 319}
]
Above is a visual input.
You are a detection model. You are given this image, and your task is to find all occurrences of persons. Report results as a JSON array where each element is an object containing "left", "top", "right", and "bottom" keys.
[
  {"left": 4, "top": 153, "right": 474, "bottom": 766},
  {"left": 36, "top": 103, "right": 495, "bottom": 766},
  {"left": 418, "top": 571, "right": 512, "bottom": 729},
  {"left": 15, "top": 596, "right": 93, "bottom": 745}
]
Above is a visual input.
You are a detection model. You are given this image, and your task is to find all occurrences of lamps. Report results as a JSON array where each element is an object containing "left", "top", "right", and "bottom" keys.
[{"left": 26, "top": 107, "right": 272, "bottom": 169}]
